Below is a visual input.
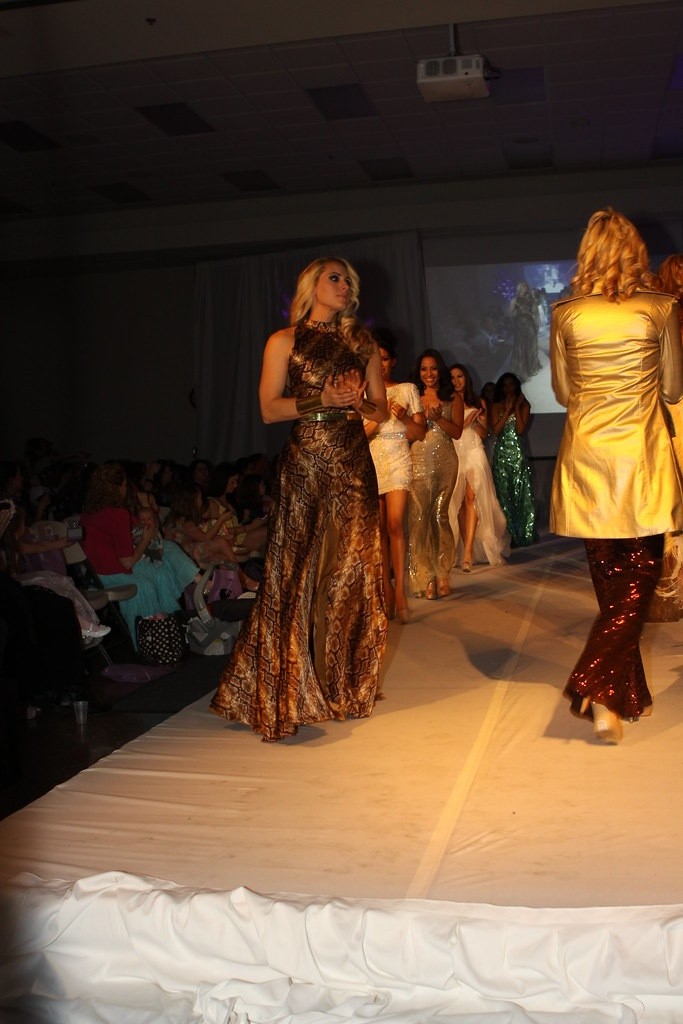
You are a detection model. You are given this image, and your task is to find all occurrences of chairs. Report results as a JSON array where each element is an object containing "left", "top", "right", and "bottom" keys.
[
  {"left": 78, "top": 588, "right": 115, "bottom": 669},
  {"left": 31, "top": 522, "right": 139, "bottom": 655}
]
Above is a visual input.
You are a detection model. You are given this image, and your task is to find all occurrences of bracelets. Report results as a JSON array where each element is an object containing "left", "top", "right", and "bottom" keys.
[
  {"left": 358, "top": 397, "right": 377, "bottom": 415},
  {"left": 294, "top": 395, "right": 325, "bottom": 417}
]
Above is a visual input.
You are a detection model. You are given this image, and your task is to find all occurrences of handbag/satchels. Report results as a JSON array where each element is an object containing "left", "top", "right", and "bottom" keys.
[{"left": 134, "top": 612, "right": 185, "bottom": 667}]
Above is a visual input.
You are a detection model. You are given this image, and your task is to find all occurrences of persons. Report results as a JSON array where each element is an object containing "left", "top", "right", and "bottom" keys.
[
  {"left": 488, "top": 371, "right": 540, "bottom": 547},
  {"left": 639, "top": 247, "right": 681, "bottom": 626},
  {"left": 439, "top": 364, "right": 511, "bottom": 575},
  {"left": 203, "top": 256, "right": 393, "bottom": 742},
  {"left": 475, "top": 378, "right": 499, "bottom": 465},
  {"left": 550, "top": 208, "right": 681, "bottom": 746},
  {"left": 2, "top": 455, "right": 286, "bottom": 664},
  {"left": 401, "top": 349, "right": 465, "bottom": 602},
  {"left": 356, "top": 339, "right": 424, "bottom": 623}
]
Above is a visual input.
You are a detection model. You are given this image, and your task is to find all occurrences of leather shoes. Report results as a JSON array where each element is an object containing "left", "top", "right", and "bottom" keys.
[{"left": 81, "top": 622, "right": 112, "bottom": 639}]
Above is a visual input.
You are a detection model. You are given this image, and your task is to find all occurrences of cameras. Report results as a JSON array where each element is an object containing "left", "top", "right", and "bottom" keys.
[{"left": 67, "top": 525, "right": 86, "bottom": 541}]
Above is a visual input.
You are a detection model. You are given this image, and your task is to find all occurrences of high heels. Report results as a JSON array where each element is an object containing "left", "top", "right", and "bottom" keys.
[
  {"left": 396, "top": 595, "right": 410, "bottom": 623},
  {"left": 423, "top": 578, "right": 452, "bottom": 600},
  {"left": 581, "top": 688, "right": 654, "bottom": 747}
]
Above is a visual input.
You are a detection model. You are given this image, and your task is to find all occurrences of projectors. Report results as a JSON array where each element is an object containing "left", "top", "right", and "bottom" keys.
[{"left": 416, "top": 54, "right": 490, "bottom": 103}]
